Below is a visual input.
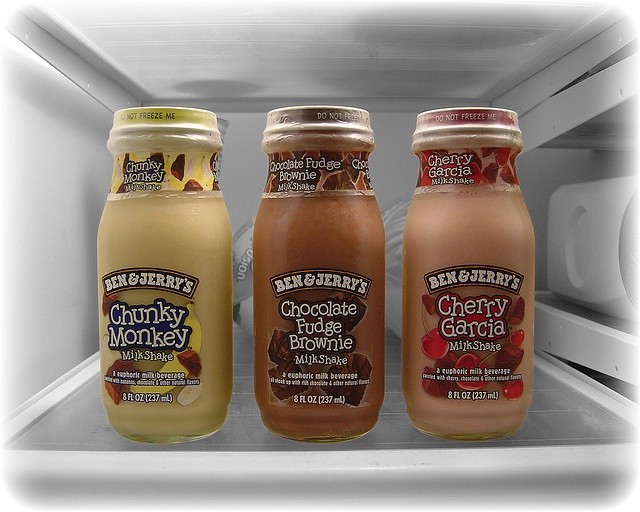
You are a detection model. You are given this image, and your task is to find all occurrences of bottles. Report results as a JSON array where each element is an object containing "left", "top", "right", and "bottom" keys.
[
  {"left": 401, "top": 106, "right": 535, "bottom": 441},
  {"left": 97, "top": 106, "right": 233, "bottom": 443},
  {"left": 252, "top": 105, "right": 385, "bottom": 442}
]
